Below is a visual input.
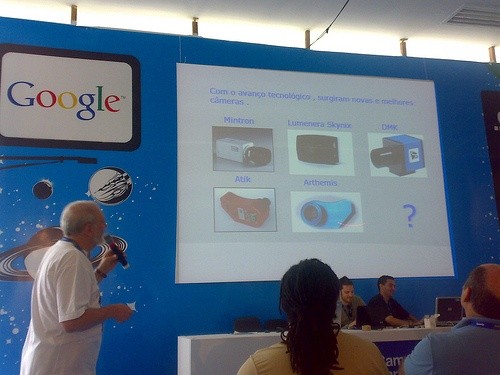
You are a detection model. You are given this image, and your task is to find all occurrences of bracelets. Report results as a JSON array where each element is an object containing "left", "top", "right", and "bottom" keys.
[{"left": 95, "top": 268, "right": 106, "bottom": 278}]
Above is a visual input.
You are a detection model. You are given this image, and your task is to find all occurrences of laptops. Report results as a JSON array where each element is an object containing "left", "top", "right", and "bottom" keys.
[
  {"left": 352, "top": 306, "right": 386, "bottom": 329},
  {"left": 435, "top": 297, "right": 466, "bottom": 327}
]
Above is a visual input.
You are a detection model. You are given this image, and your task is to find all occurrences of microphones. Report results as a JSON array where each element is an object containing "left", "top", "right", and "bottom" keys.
[{"left": 104, "top": 234, "right": 128, "bottom": 268}]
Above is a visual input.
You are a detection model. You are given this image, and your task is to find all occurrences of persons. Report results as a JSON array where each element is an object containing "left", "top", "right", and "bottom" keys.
[
  {"left": 404, "top": 264, "right": 500, "bottom": 375},
  {"left": 20, "top": 200, "right": 132, "bottom": 375},
  {"left": 237, "top": 259, "right": 392, "bottom": 375},
  {"left": 336, "top": 276, "right": 366, "bottom": 329},
  {"left": 369, "top": 276, "right": 424, "bottom": 327}
]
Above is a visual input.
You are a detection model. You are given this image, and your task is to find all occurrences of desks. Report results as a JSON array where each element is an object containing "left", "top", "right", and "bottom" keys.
[{"left": 176, "top": 324, "right": 455, "bottom": 375}]
